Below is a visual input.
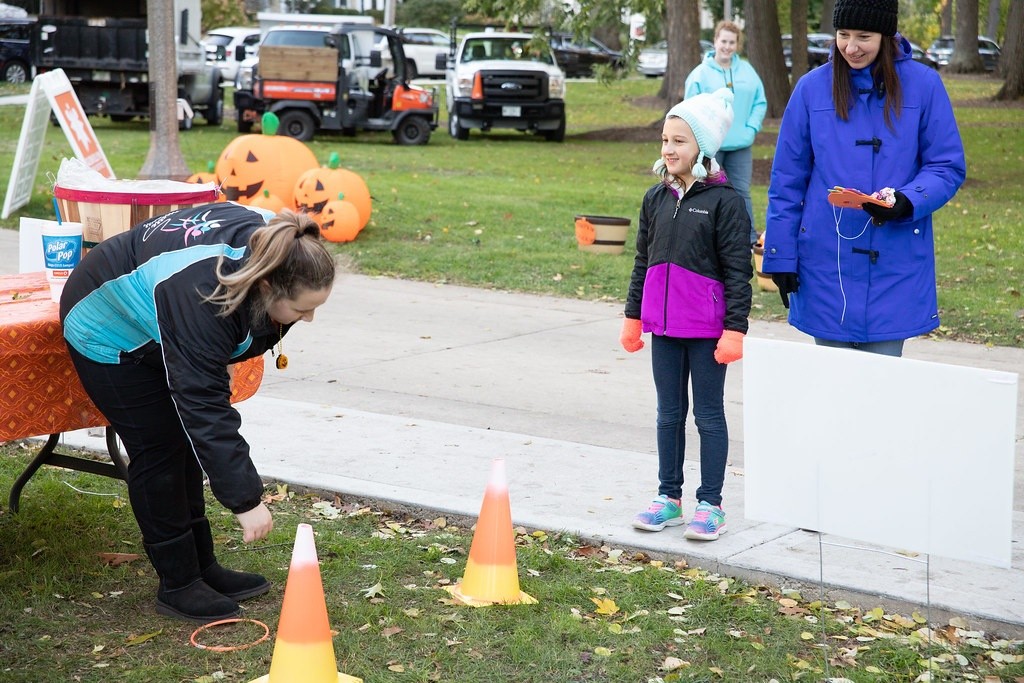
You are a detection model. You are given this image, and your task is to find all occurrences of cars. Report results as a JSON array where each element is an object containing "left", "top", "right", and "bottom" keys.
[
  {"left": 203, "top": 27, "right": 261, "bottom": 90},
  {"left": 0, "top": 17, "right": 38, "bottom": 85},
  {"left": 926, "top": 35, "right": 1003, "bottom": 72},
  {"left": 910, "top": 41, "right": 941, "bottom": 71},
  {"left": 637, "top": 41, "right": 715, "bottom": 78},
  {"left": 781, "top": 33, "right": 838, "bottom": 73},
  {"left": 371, "top": 26, "right": 457, "bottom": 80},
  {"left": 542, "top": 30, "right": 629, "bottom": 79}
]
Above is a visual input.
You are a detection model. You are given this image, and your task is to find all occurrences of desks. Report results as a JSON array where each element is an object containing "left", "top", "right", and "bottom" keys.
[{"left": 0, "top": 272, "right": 264, "bottom": 513}]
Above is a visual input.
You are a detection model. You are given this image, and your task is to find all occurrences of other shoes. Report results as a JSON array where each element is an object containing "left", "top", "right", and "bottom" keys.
[{"left": 750, "top": 242, "right": 757, "bottom": 253}]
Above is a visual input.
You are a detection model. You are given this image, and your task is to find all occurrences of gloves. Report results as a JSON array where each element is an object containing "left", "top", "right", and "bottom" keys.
[
  {"left": 620, "top": 317, "right": 644, "bottom": 352},
  {"left": 714, "top": 331, "right": 744, "bottom": 363},
  {"left": 862, "top": 191, "right": 914, "bottom": 226},
  {"left": 772, "top": 272, "right": 801, "bottom": 309}
]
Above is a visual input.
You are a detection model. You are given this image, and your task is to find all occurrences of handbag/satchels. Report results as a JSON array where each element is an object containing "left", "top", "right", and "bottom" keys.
[{"left": 753, "top": 230, "right": 779, "bottom": 291}]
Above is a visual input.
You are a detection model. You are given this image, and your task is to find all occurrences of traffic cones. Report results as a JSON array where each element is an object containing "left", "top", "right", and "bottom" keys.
[
  {"left": 245, "top": 521, "right": 363, "bottom": 683},
  {"left": 439, "top": 458, "right": 541, "bottom": 610}
]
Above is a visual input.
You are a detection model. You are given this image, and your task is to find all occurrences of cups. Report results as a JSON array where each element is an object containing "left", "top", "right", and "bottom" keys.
[{"left": 39, "top": 221, "right": 84, "bottom": 303}]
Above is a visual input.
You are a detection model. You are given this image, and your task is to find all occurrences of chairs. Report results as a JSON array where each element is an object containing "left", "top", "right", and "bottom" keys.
[{"left": 472, "top": 45, "right": 485, "bottom": 58}]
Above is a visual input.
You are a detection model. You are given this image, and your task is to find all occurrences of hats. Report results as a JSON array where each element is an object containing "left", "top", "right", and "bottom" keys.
[
  {"left": 832, "top": 0, "right": 899, "bottom": 36},
  {"left": 653, "top": 88, "right": 735, "bottom": 183}
]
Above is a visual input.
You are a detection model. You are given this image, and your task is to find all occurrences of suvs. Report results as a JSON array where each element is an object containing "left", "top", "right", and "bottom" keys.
[{"left": 442, "top": 17, "right": 568, "bottom": 143}]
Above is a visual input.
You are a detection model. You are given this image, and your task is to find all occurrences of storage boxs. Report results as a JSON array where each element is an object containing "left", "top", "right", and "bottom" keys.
[{"left": 259, "top": 46, "right": 338, "bottom": 81}]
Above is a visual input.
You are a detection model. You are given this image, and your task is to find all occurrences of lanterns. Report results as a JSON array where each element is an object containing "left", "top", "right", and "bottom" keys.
[
  {"left": 320, "top": 192, "right": 359, "bottom": 242},
  {"left": 186, "top": 160, "right": 227, "bottom": 203},
  {"left": 251, "top": 190, "right": 283, "bottom": 214},
  {"left": 214, "top": 113, "right": 319, "bottom": 212},
  {"left": 293, "top": 151, "right": 371, "bottom": 231}
]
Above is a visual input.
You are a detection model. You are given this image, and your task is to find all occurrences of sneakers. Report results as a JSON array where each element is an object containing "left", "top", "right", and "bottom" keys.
[
  {"left": 632, "top": 494, "right": 685, "bottom": 531},
  {"left": 682, "top": 501, "right": 726, "bottom": 540}
]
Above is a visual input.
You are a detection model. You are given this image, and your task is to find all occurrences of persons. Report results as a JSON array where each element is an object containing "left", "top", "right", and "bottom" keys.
[
  {"left": 684, "top": 20, "right": 767, "bottom": 247},
  {"left": 762, "top": 0, "right": 966, "bottom": 357},
  {"left": 619, "top": 87, "right": 753, "bottom": 539},
  {"left": 59, "top": 201, "right": 334, "bottom": 621}
]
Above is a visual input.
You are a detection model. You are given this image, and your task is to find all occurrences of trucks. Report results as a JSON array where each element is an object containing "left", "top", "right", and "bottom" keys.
[
  {"left": 232, "top": 12, "right": 380, "bottom": 134},
  {"left": 36, "top": 0, "right": 228, "bottom": 130}
]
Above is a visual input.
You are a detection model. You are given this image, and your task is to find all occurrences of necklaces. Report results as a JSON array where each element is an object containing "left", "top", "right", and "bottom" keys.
[{"left": 276, "top": 323, "right": 288, "bottom": 369}]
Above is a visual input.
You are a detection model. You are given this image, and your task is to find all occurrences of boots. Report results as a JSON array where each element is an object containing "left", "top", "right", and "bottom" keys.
[
  {"left": 142, "top": 529, "right": 244, "bottom": 623},
  {"left": 191, "top": 517, "right": 269, "bottom": 601}
]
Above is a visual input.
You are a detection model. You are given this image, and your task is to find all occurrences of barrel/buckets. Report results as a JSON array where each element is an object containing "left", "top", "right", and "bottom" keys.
[
  {"left": 752, "top": 243, "right": 780, "bottom": 292},
  {"left": 574, "top": 214, "right": 631, "bottom": 255},
  {"left": 46, "top": 170, "right": 229, "bottom": 260}
]
